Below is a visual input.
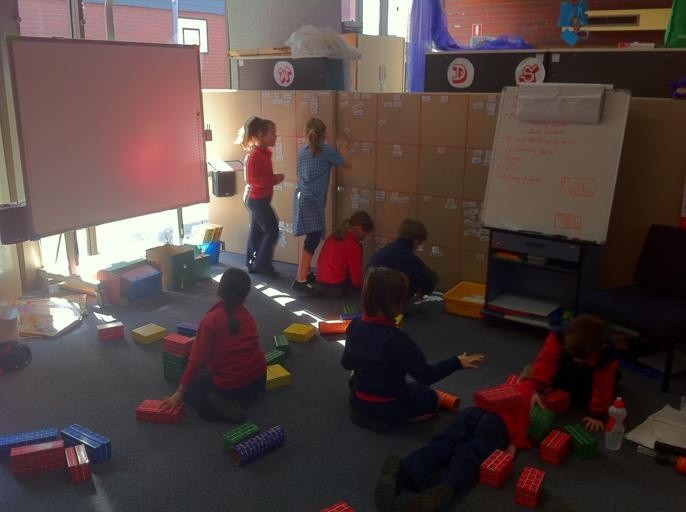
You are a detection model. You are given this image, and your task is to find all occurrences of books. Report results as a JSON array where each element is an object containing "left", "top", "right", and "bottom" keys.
[{"left": 624, "top": 403, "right": 686, "bottom": 452}]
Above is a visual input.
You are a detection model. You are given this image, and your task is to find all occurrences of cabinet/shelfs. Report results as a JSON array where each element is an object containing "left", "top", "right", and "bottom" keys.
[
  {"left": 486, "top": 229, "right": 584, "bottom": 333},
  {"left": 343, "top": 32, "right": 405, "bottom": 92},
  {"left": 258, "top": 93, "right": 501, "bottom": 294}
]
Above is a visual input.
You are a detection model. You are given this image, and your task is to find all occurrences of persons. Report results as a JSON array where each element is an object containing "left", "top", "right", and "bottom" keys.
[
  {"left": 291, "top": 118, "right": 352, "bottom": 296},
  {"left": 233, "top": 115, "right": 284, "bottom": 278},
  {"left": 159, "top": 267, "right": 267, "bottom": 414},
  {"left": 315, "top": 211, "right": 364, "bottom": 298},
  {"left": 363, "top": 217, "right": 437, "bottom": 300},
  {"left": 526, "top": 313, "right": 622, "bottom": 433},
  {"left": 373, "top": 384, "right": 560, "bottom": 512},
  {"left": 339, "top": 267, "right": 485, "bottom": 422}
]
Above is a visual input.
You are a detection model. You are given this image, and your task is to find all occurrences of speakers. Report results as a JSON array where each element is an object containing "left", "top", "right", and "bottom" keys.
[
  {"left": 206, "top": 160, "right": 235, "bottom": 197},
  {"left": 1, "top": 202, "right": 29, "bottom": 245}
]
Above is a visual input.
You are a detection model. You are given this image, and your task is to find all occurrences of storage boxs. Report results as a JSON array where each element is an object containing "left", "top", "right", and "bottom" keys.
[
  {"left": 184, "top": 239, "right": 219, "bottom": 264},
  {"left": 444, "top": 281, "right": 485, "bottom": 317},
  {"left": 113, "top": 260, "right": 162, "bottom": 302},
  {"left": 194, "top": 254, "right": 211, "bottom": 281},
  {"left": 548, "top": 47, "right": 686, "bottom": 98},
  {"left": 146, "top": 245, "right": 194, "bottom": 289},
  {"left": 238, "top": 58, "right": 343, "bottom": 91},
  {"left": 424, "top": 52, "right": 548, "bottom": 93}
]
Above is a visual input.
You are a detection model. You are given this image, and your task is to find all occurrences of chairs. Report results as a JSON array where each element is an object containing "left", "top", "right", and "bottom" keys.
[{"left": 585, "top": 224, "right": 686, "bottom": 393}]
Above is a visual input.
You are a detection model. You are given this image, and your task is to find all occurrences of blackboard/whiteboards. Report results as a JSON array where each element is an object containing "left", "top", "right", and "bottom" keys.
[
  {"left": 479, "top": 86, "right": 632, "bottom": 245},
  {"left": 4, "top": 34, "right": 210, "bottom": 240}
]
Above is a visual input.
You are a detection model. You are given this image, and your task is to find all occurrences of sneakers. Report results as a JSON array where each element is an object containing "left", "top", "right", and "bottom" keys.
[
  {"left": 251, "top": 265, "right": 281, "bottom": 278},
  {"left": 400, "top": 483, "right": 457, "bottom": 512},
  {"left": 202, "top": 391, "right": 248, "bottom": 424},
  {"left": 372, "top": 454, "right": 402, "bottom": 512},
  {"left": 291, "top": 279, "right": 322, "bottom": 298},
  {"left": 308, "top": 273, "right": 317, "bottom": 282},
  {"left": 248, "top": 264, "right": 275, "bottom": 273}
]
